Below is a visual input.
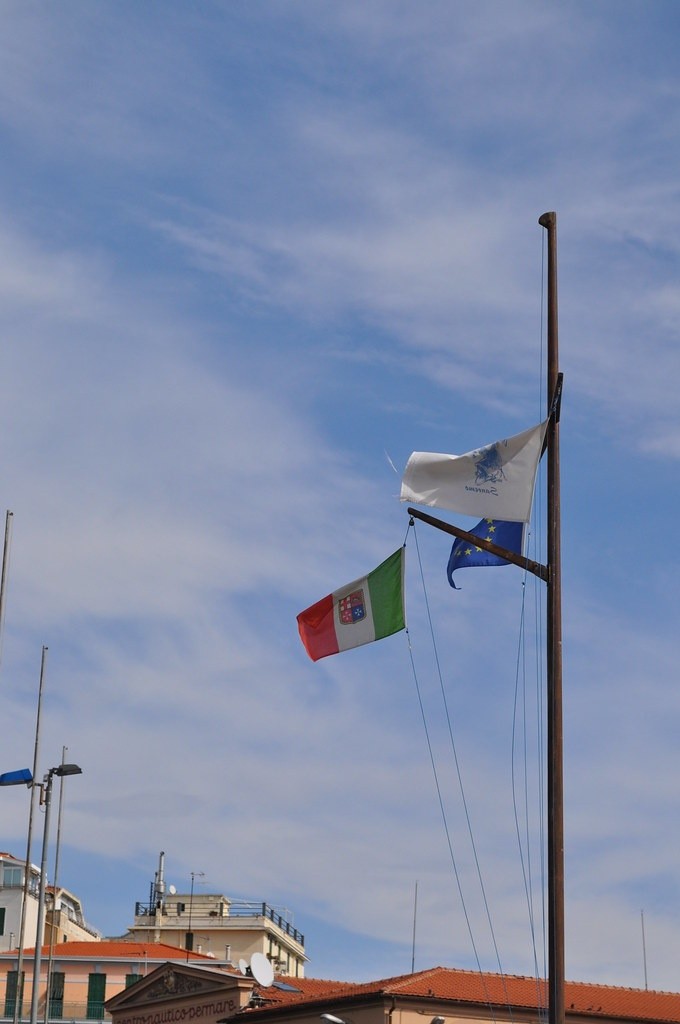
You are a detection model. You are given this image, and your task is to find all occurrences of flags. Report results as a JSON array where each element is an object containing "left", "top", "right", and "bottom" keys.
[
  {"left": 400, "top": 418, "right": 548, "bottom": 522},
  {"left": 447, "top": 518, "right": 523, "bottom": 590},
  {"left": 296, "top": 547, "right": 406, "bottom": 662}
]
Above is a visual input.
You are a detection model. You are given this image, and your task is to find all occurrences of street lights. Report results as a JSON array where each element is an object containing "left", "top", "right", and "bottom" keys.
[{"left": 0, "top": 763, "right": 86, "bottom": 1024}]
